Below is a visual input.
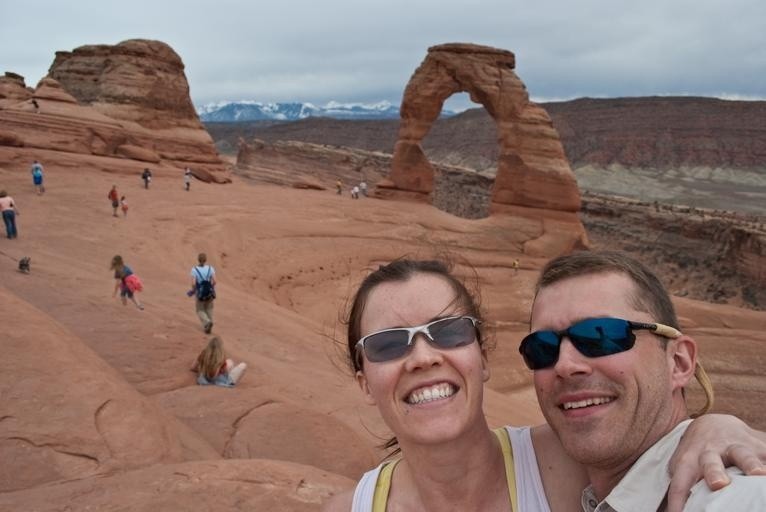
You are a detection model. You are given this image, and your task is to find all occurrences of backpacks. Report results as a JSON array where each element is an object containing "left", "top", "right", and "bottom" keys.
[{"left": 193, "top": 266, "right": 216, "bottom": 301}]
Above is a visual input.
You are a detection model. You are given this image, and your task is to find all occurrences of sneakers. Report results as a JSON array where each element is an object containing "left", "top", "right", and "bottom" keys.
[{"left": 203, "top": 321, "right": 213, "bottom": 334}]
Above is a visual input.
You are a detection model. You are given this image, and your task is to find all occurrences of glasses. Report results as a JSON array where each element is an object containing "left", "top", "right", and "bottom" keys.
[
  {"left": 516, "top": 316, "right": 683, "bottom": 371},
  {"left": 353, "top": 315, "right": 483, "bottom": 368}
]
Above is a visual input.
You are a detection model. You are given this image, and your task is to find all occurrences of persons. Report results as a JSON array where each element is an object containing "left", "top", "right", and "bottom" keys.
[
  {"left": 191, "top": 254, "right": 216, "bottom": 333},
  {"left": 31, "top": 160, "right": 45, "bottom": 192},
  {"left": 142, "top": 168, "right": 151, "bottom": 189},
  {"left": 519, "top": 250, "right": 766, "bottom": 511},
  {"left": 184, "top": 166, "right": 193, "bottom": 190},
  {"left": 109, "top": 256, "right": 145, "bottom": 310},
  {"left": 325, "top": 253, "right": 766, "bottom": 512},
  {"left": 336, "top": 179, "right": 368, "bottom": 199},
  {"left": 120, "top": 196, "right": 128, "bottom": 219},
  {"left": 191, "top": 336, "right": 246, "bottom": 387},
  {"left": 33, "top": 166, "right": 42, "bottom": 194},
  {"left": 108, "top": 185, "right": 119, "bottom": 217},
  {"left": 0, "top": 190, "right": 20, "bottom": 239}
]
